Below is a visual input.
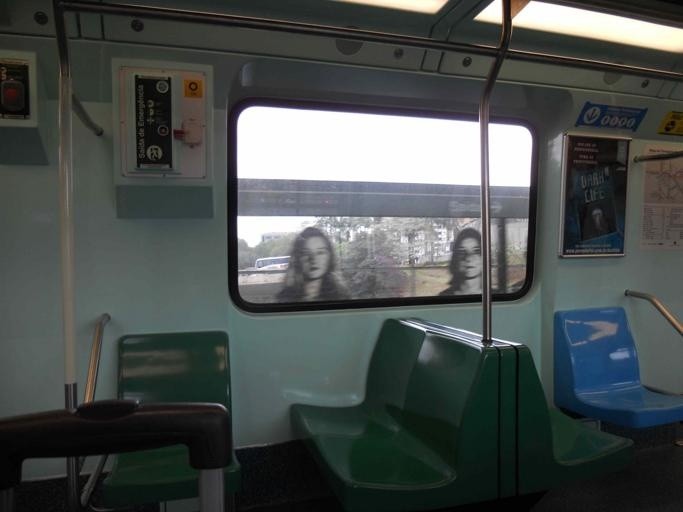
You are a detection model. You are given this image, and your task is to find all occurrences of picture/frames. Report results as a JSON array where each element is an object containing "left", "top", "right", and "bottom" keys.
[{"left": 558, "top": 131, "right": 633, "bottom": 259}]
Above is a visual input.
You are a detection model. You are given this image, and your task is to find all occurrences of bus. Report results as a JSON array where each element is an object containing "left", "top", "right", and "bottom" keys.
[{"left": 255, "top": 256, "right": 291, "bottom": 271}]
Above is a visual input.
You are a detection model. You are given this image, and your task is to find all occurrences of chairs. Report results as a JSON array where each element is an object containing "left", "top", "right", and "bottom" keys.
[
  {"left": 100, "top": 331, "right": 244, "bottom": 511},
  {"left": 553, "top": 306, "right": 682, "bottom": 449}
]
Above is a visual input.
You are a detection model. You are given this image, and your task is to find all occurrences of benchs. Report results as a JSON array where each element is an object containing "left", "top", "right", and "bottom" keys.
[{"left": 288, "top": 318, "right": 636, "bottom": 512}]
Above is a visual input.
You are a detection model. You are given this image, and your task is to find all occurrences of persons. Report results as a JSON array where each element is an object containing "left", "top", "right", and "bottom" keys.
[
  {"left": 439, "top": 227, "right": 498, "bottom": 296},
  {"left": 274, "top": 227, "right": 352, "bottom": 303}
]
